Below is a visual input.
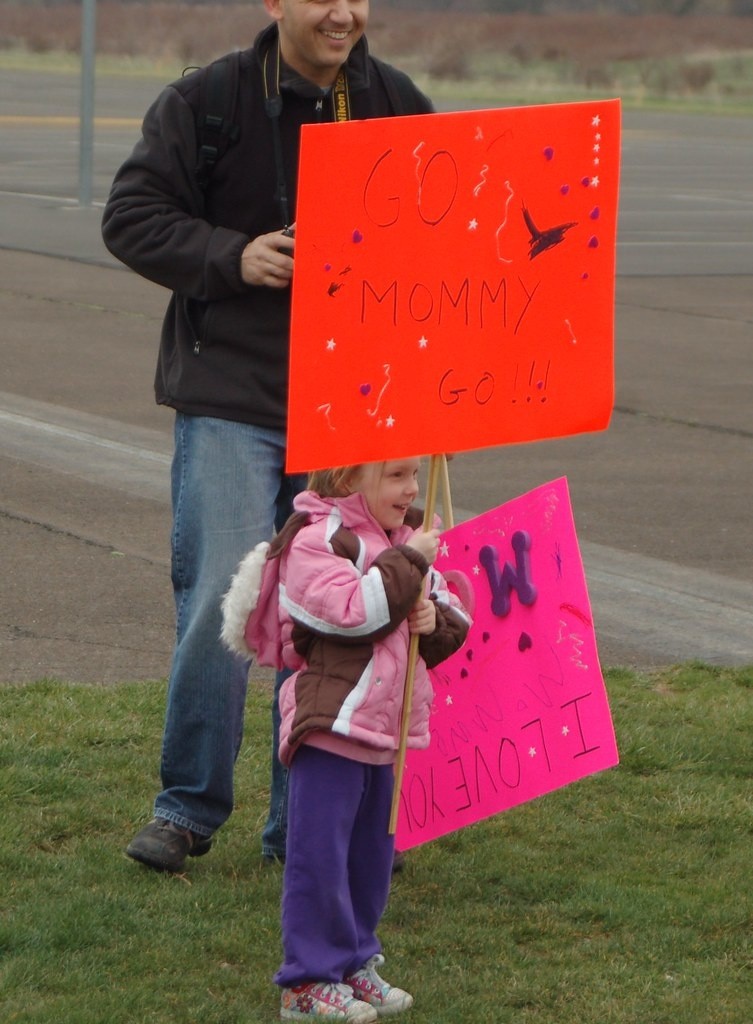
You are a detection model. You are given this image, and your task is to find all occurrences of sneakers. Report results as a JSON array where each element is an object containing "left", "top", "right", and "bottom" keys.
[
  {"left": 339, "top": 953, "right": 414, "bottom": 1013},
  {"left": 280, "top": 983, "right": 378, "bottom": 1023},
  {"left": 279, "top": 848, "right": 405, "bottom": 873},
  {"left": 126, "top": 816, "right": 213, "bottom": 869}
]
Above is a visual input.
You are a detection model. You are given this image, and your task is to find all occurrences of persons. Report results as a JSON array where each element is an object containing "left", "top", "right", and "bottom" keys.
[
  {"left": 101, "top": 1, "right": 433, "bottom": 873},
  {"left": 221, "top": 455, "right": 473, "bottom": 1022}
]
